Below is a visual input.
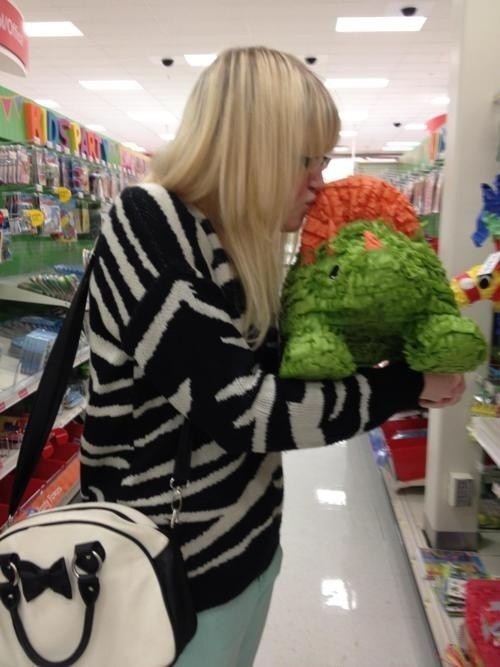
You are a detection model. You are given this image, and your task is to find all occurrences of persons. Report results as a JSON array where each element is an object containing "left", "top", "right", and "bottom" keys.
[{"left": 76, "top": 42, "right": 467, "bottom": 666}]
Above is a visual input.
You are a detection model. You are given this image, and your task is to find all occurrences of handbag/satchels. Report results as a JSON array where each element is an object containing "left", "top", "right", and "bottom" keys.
[{"left": 0, "top": 502, "right": 197, "bottom": 667}]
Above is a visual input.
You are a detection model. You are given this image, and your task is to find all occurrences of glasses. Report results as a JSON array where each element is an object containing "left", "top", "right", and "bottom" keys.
[{"left": 302, "top": 155, "right": 331, "bottom": 169}]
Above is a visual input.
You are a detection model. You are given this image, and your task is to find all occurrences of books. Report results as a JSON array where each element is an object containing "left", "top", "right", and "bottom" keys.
[{"left": 418, "top": 545, "right": 490, "bottom": 617}]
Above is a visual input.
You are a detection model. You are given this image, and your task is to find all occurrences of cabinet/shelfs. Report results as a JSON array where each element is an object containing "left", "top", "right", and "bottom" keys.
[{"left": 0, "top": 274, "right": 152, "bottom": 543}]
